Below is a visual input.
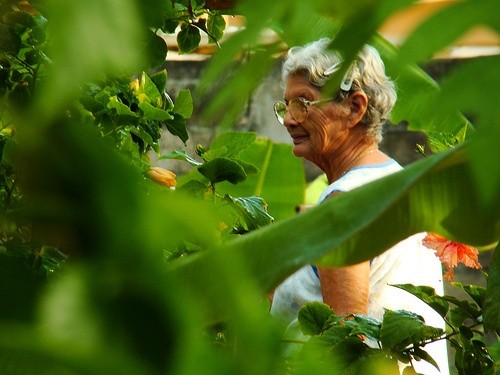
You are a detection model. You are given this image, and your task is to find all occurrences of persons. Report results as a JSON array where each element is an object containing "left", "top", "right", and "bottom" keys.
[{"left": 269, "top": 38, "right": 450, "bottom": 375}]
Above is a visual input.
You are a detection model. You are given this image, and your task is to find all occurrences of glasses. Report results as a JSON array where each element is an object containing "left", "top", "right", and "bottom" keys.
[{"left": 273, "top": 97, "right": 337, "bottom": 127}]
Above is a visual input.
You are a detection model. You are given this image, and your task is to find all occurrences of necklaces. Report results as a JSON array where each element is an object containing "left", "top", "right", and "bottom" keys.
[{"left": 336, "top": 149, "right": 378, "bottom": 179}]
